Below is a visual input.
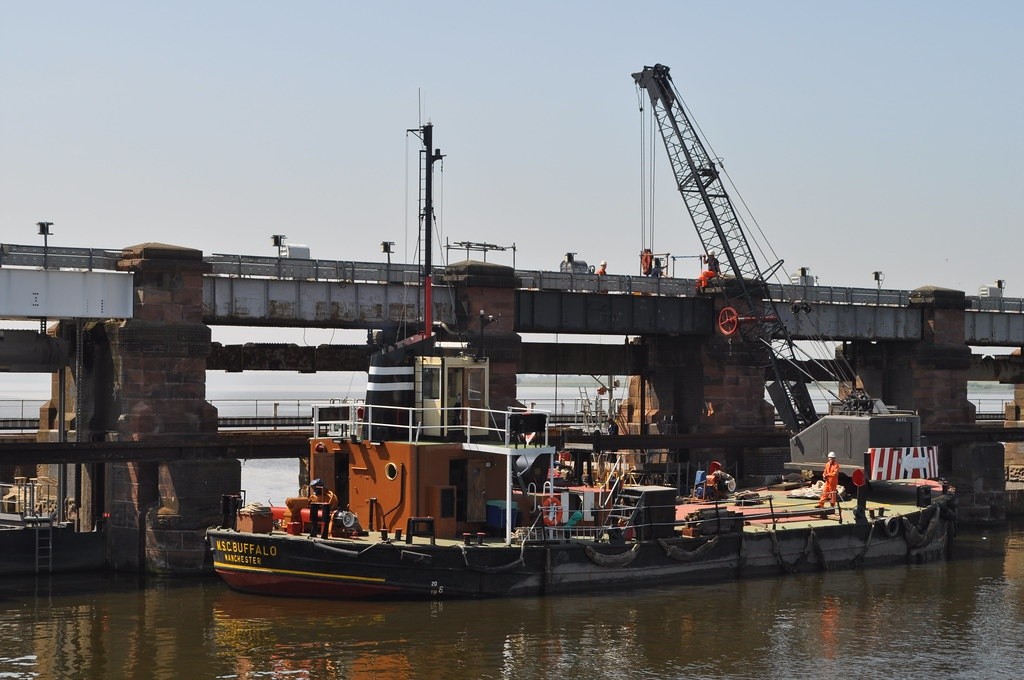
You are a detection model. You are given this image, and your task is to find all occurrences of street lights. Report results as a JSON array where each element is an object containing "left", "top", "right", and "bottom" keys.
[
  {"left": 381, "top": 241, "right": 394, "bottom": 320},
  {"left": 271, "top": 235, "right": 286, "bottom": 280},
  {"left": 872, "top": 272, "right": 883, "bottom": 306},
  {"left": 37, "top": 222, "right": 53, "bottom": 271},
  {"left": 995, "top": 280, "right": 1006, "bottom": 312},
  {"left": 798, "top": 268, "right": 809, "bottom": 302}
]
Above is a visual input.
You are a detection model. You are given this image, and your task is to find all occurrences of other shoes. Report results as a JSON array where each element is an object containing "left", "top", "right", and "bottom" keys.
[
  {"left": 699, "top": 287, "right": 706, "bottom": 289},
  {"left": 815, "top": 505, "right": 820, "bottom": 508},
  {"left": 830, "top": 505, "right": 835, "bottom": 508}
]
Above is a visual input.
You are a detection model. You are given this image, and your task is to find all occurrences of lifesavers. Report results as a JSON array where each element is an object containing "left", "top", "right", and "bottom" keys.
[{"left": 543, "top": 496, "right": 563, "bottom": 526}]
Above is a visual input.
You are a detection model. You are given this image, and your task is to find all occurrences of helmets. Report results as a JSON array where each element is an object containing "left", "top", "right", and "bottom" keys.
[
  {"left": 600, "top": 261, "right": 608, "bottom": 265},
  {"left": 707, "top": 250, "right": 716, "bottom": 256},
  {"left": 827, "top": 452, "right": 836, "bottom": 458}
]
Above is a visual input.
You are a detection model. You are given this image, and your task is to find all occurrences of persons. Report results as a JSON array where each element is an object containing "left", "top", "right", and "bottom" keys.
[
  {"left": 595, "top": 262, "right": 608, "bottom": 294},
  {"left": 607, "top": 417, "right": 619, "bottom": 437},
  {"left": 696, "top": 253, "right": 721, "bottom": 287},
  {"left": 816, "top": 451, "right": 839, "bottom": 509}
]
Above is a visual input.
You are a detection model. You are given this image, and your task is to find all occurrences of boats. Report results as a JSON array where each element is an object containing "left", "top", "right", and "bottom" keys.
[{"left": 202, "top": 88, "right": 958, "bottom": 601}]
